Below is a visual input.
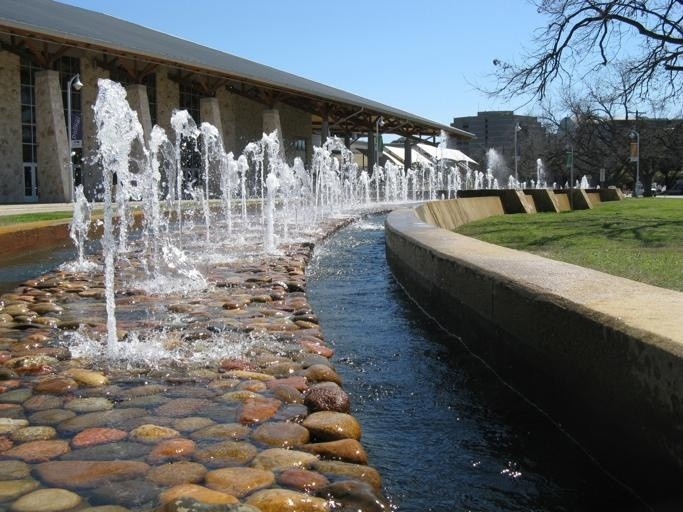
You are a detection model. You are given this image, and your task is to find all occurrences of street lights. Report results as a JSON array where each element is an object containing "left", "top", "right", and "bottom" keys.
[
  {"left": 515, "top": 122, "right": 521, "bottom": 179},
  {"left": 566, "top": 141, "right": 573, "bottom": 210},
  {"left": 629, "top": 130, "right": 639, "bottom": 195},
  {"left": 67, "top": 73, "right": 83, "bottom": 203},
  {"left": 377, "top": 116, "right": 384, "bottom": 167}
]
